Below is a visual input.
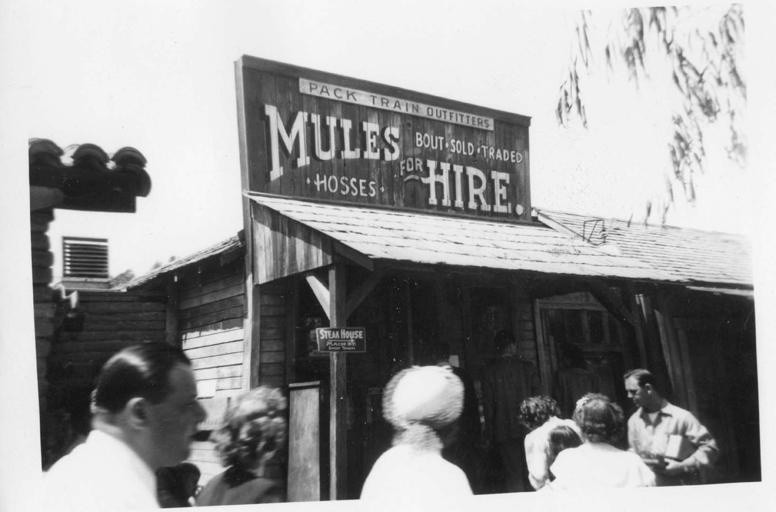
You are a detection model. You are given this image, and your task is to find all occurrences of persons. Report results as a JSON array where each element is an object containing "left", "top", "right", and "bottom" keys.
[
  {"left": 194, "top": 384, "right": 287, "bottom": 507},
  {"left": 156, "top": 462, "right": 201, "bottom": 508},
  {"left": 360, "top": 365, "right": 474, "bottom": 500},
  {"left": 43, "top": 339, "right": 208, "bottom": 508},
  {"left": 518, "top": 369, "right": 720, "bottom": 492}
]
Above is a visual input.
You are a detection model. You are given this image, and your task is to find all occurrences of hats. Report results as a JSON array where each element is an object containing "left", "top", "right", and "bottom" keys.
[{"left": 496, "top": 331, "right": 515, "bottom": 344}]
